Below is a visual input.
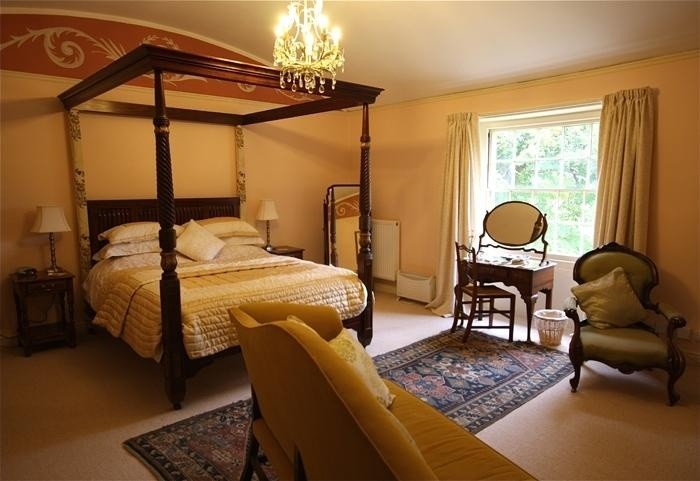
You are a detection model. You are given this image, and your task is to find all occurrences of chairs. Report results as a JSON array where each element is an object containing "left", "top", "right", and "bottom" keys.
[{"left": 451, "top": 244, "right": 519, "bottom": 345}]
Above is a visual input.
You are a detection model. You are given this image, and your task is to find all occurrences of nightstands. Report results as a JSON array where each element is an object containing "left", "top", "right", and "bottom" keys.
[
  {"left": 8, "top": 269, "right": 82, "bottom": 356},
  {"left": 263, "top": 242, "right": 307, "bottom": 261}
]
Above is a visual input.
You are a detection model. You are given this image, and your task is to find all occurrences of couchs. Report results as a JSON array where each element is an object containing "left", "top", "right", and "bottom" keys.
[
  {"left": 230, "top": 297, "right": 540, "bottom": 481},
  {"left": 563, "top": 243, "right": 690, "bottom": 407}
]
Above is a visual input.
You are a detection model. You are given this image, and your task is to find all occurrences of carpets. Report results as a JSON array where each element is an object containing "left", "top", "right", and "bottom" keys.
[{"left": 124, "top": 323, "right": 576, "bottom": 481}]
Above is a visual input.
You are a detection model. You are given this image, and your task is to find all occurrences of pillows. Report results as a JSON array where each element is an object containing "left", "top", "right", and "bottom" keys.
[
  {"left": 325, "top": 326, "right": 395, "bottom": 409},
  {"left": 176, "top": 220, "right": 224, "bottom": 262},
  {"left": 220, "top": 236, "right": 266, "bottom": 247},
  {"left": 181, "top": 215, "right": 258, "bottom": 238},
  {"left": 568, "top": 266, "right": 649, "bottom": 331},
  {"left": 92, "top": 242, "right": 168, "bottom": 262},
  {"left": 94, "top": 224, "right": 186, "bottom": 244}
]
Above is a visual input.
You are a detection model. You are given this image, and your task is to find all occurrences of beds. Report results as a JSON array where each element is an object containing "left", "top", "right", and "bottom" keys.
[{"left": 85, "top": 192, "right": 370, "bottom": 410}]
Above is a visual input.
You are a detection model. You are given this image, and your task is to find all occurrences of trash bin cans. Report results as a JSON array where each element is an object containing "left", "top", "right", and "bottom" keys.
[{"left": 534, "top": 309, "right": 568, "bottom": 346}]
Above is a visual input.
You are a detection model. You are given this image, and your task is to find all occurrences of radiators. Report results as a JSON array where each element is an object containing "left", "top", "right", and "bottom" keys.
[{"left": 370, "top": 218, "right": 401, "bottom": 282}]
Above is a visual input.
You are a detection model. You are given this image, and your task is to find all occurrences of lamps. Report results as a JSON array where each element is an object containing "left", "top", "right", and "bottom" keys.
[
  {"left": 255, "top": 197, "right": 285, "bottom": 249},
  {"left": 273, "top": 0, "right": 347, "bottom": 94},
  {"left": 29, "top": 203, "right": 81, "bottom": 272}
]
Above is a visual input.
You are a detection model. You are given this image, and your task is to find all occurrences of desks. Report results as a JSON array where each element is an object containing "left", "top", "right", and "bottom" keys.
[{"left": 453, "top": 250, "right": 558, "bottom": 347}]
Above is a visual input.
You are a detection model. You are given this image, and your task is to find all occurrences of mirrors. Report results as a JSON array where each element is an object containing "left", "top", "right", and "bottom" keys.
[
  {"left": 322, "top": 182, "right": 367, "bottom": 280},
  {"left": 474, "top": 199, "right": 549, "bottom": 265}
]
